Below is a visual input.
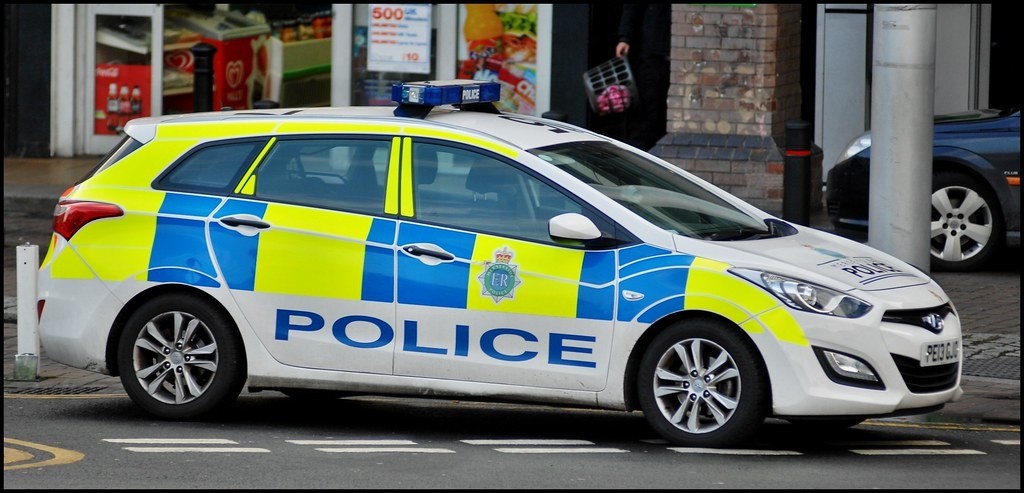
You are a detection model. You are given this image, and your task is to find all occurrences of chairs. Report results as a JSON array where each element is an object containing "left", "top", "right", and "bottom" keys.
[{"left": 411, "top": 142, "right": 518, "bottom": 203}]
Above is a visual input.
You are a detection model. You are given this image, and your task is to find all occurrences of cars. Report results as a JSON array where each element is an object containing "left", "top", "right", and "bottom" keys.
[
  {"left": 17, "top": 80, "right": 964, "bottom": 444},
  {"left": 825, "top": 104, "right": 1021, "bottom": 272}
]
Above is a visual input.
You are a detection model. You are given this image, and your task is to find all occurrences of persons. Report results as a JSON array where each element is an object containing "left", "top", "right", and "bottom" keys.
[{"left": 615, "top": 3, "right": 672, "bottom": 148}]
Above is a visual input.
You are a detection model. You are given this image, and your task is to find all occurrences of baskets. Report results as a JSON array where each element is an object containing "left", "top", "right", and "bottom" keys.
[{"left": 582, "top": 50, "right": 641, "bottom": 116}]
